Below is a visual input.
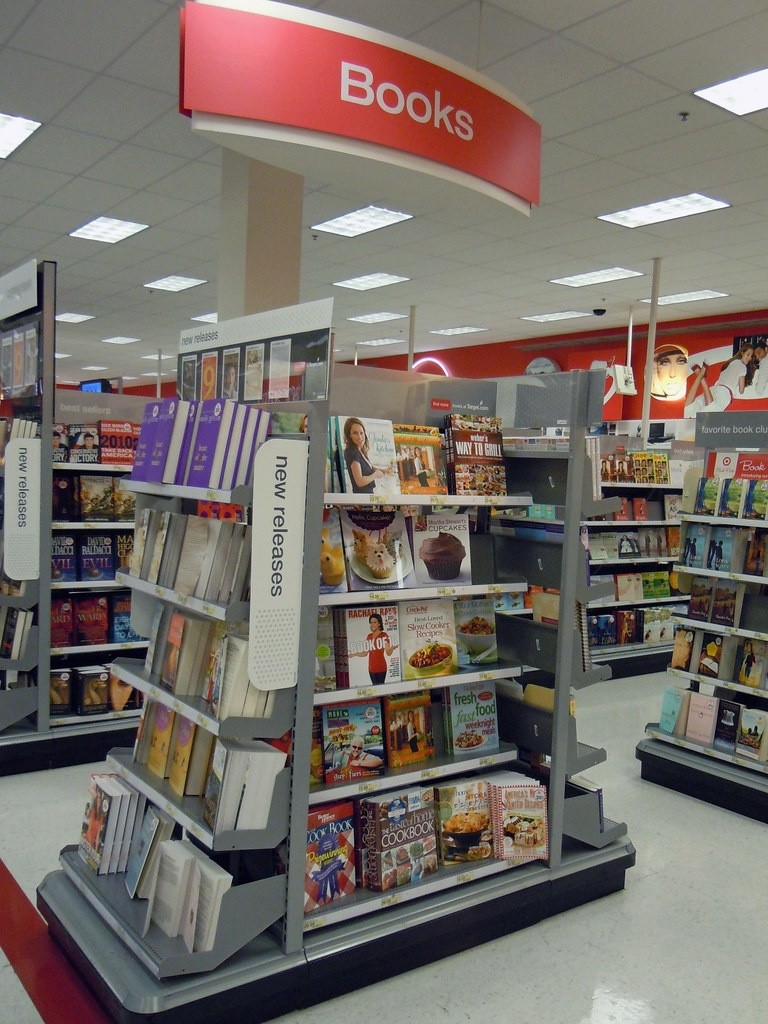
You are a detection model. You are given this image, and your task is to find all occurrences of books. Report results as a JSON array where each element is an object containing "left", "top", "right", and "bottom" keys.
[
  {"left": 627, "top": 452, "right": 656, "bottom": 484},
  {"left": 654, "top": 454, "right": 669, "bottom": 484},
  {"left": 0, "top": 397, "right": 551, "bottom": 953},
  {"left": 577, "top": 467, "right": 768, "bottom": 761}
]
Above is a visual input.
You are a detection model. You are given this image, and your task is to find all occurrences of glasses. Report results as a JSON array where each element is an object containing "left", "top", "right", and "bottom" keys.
[{"left": 352, "top": 745, "right": 363, "bottom": 750}]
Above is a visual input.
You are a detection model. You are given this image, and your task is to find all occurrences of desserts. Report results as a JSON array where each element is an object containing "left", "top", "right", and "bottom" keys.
[
  {"left": 419, "top": 532, "right": 466, "bottom": 580},
  {"left": 352, "top": 529, "right": 402, "bottom": 579},
  {"left": 321, "top": 527, "right": 344, "bottom": 585}
]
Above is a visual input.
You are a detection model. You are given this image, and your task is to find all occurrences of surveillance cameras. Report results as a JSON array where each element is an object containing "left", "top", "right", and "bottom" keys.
[{"left": 593, "top": 309, "right": 606, "bottom": 316}]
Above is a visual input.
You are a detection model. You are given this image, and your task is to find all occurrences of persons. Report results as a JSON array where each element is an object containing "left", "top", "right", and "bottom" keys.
[
  {"left": 333, "top": 737, "right": 383, "bottom": 769},
  {"left": 406, "top": 708, "right": 420, "bottom": 753},
  {"left": 654, "top": 461, "right": 668, "bottom": 484},
  {"left": 653, "top": 350, "right": 687, "bottom": 398},
  {"left": 601, "top": 460, "right": 609, "bottom": 482},
  {"left": 344, "top": 417, "right": 393, "bottom": 494},
  {"left": 684, "top": 341, "right": 768, "bottom": 419},
  {"left": 413, "top": 446, "right": 429, "bottom": 487},
  {"left": 223, "top": 359, "right": 237, "bottom": 398},
  {"left": 349, "top": 613, "right": 400, "bottom": 685},
  {"left": 77, "top": 433, "right": 100, "bottom": 463},
  {"left": 53, "top": 429, "right": 68, "bottom": 463},
  {"left": 617, "top": 461, "right": 631, "bottom": 479},
  {"left": 635, "top": 459, "right": 655, "bottom": 484}
]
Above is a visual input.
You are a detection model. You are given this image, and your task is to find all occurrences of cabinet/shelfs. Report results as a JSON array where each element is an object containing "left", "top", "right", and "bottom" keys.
[{"left": 0, "top": 258, "right": 768, "bottom": 1024}]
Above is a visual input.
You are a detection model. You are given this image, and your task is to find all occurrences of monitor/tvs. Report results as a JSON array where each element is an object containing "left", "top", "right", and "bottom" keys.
[
  {"left": 80, "top": 378, "right": 112, "bottom": 393},
  {"left": 649, "top": 423, "right": 665, "bottom": 438}
]
